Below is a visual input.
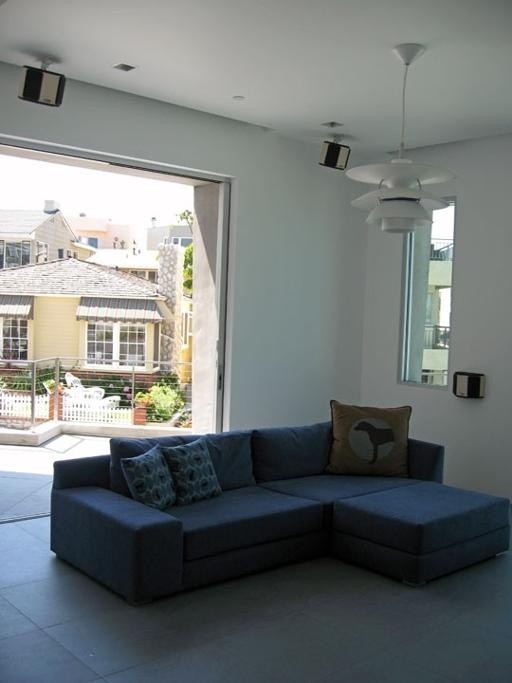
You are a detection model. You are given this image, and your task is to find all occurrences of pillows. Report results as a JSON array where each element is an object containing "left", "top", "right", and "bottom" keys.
[
  {"left": 123, "top": 437, "right": 224, "bottom": 510},
  {"left": 324, "top": 397, "right": 410, "bottom": 477}
]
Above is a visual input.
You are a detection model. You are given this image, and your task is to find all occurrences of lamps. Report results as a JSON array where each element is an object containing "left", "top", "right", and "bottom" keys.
[{"left": 346, "top": 43, "right": 456, "bottom": 234}]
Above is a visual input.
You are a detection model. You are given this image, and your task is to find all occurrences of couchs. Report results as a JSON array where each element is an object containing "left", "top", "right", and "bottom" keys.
[{"left": 51, "top": 417, "right": 508, "bottom": 608}]
